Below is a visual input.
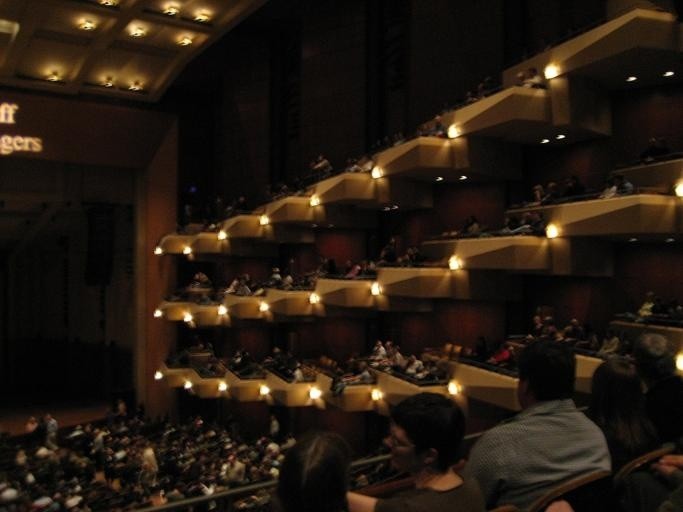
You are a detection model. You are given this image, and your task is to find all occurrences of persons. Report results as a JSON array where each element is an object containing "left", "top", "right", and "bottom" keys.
[
  {"left": 347, "top": 331, "right": 683, "bottom": 512},
  {"left": 1, "top": 397, "right": 372, "bottom": 511},
  {"left": 172, "top": 66, "right": 683, "bottom": 394}
]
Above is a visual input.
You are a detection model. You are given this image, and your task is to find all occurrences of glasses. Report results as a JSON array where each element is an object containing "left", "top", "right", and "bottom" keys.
[{"left": 390, "top": 428, "right": 412, "bottom": 448}]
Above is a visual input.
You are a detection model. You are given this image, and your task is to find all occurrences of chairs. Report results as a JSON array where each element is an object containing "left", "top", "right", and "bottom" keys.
[{"left": 0, "top": 79, "right": 681, "bottom": 510}]
